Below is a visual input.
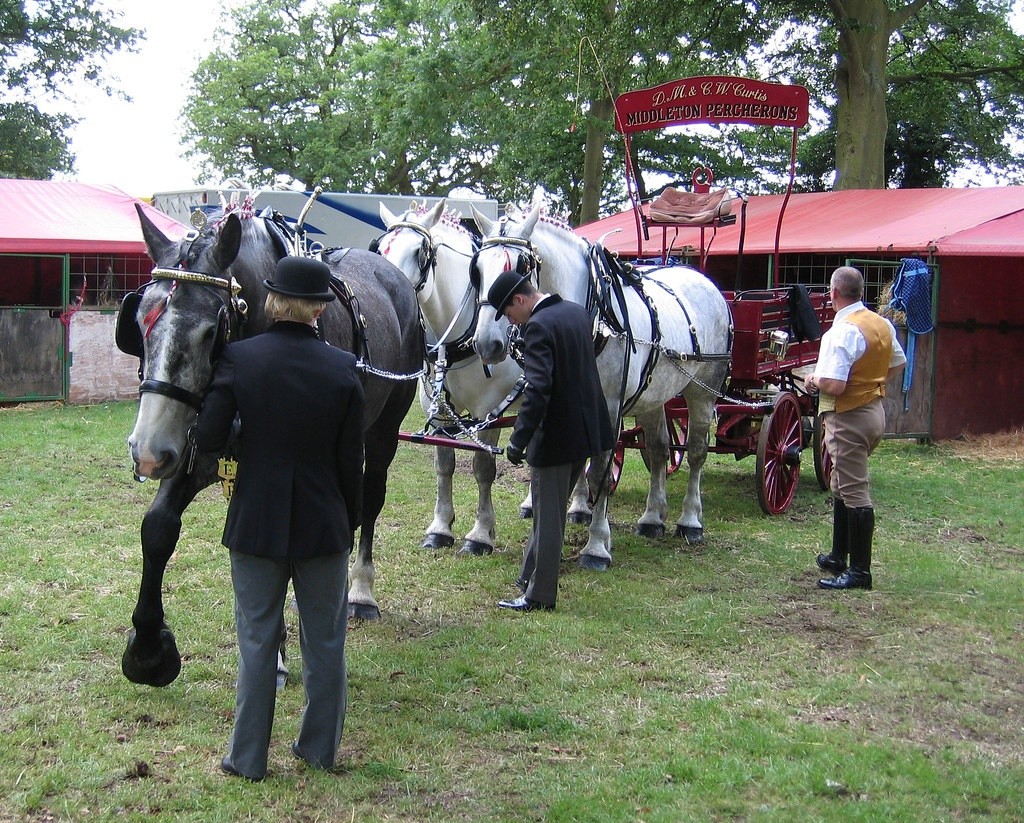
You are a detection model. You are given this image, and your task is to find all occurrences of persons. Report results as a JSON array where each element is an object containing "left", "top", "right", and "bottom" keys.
[
  {"left": 803, "top": 267, "right": 909, "bottom": 592},
  {"left": 189, "top": 255, "right": 376, "bottom": 785},
  {"left": 486, "top": 272, "right": 616, "bottom": 613}
]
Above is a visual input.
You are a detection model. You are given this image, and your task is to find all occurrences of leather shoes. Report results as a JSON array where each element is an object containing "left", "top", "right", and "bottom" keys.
[{"left": 497, "top": 576, "right": 556, "bottom": 612}]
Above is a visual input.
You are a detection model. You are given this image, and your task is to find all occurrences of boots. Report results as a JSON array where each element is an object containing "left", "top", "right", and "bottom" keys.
[{"left": 816, "top": 498, "right": 874, "bottom": 591}]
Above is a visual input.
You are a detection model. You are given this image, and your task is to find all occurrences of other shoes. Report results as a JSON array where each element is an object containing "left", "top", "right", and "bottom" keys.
[
  {"left": 293, "top": 740, "right": 335, "bottom": 772},
  {"left": 221, "top": 754, "right": 264, "bottom": 780}
]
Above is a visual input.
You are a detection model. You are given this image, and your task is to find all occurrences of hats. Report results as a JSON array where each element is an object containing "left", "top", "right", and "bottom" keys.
[
  {"left": 262, "top": 255, "right": 335, "bottom": 300},
  {"left": 487, "top": 269, "right": 532, "bottom": 321}
]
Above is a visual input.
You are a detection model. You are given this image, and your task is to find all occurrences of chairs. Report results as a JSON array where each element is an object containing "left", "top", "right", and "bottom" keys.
[{"left": 645, "top": 167, "right": 738, "bottom": 275}]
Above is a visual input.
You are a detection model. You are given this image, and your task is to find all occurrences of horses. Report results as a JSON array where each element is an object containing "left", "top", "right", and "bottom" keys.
[
  {"left": 115, "top": 203, "right": 424, "bottom": 689},
  {"left": 466, "top": 197, "right": 735, "bottom": 574},
  {"left": 368, "top": 198, "right": 611, "bottom": 557}
]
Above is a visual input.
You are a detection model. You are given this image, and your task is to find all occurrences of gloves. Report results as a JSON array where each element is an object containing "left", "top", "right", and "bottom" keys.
[{"left": 507, "top": 446, "right": 529, "bottom": 465}]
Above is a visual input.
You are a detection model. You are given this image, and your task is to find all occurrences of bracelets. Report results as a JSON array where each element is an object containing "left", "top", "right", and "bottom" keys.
[{"left": 810, "top": 373, "right": 816, "bottom": 388}]
